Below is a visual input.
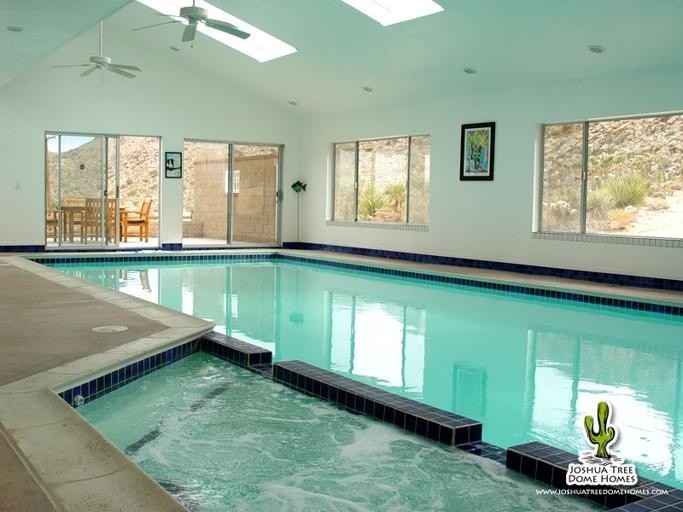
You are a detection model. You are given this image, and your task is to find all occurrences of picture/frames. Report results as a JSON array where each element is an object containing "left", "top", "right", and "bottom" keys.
[
  {"left": 165, "top": 152, "right": 182, "bottom": 178},
  {"left": 460, "top": 122, "right": 495, "bottom": 181}
]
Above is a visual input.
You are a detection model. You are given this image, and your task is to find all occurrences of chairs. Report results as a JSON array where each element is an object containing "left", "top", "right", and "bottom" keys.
[{"left": 47, "top": 198, "right": 153, "bottom": 246}]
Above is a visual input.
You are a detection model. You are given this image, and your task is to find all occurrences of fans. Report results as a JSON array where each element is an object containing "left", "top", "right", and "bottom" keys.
[
  {"left": 51, "top": 21, "right": 141, "bottom": 79},
  {"left": 131, "top": 0, "right": 251, "bottom": 42}
]
